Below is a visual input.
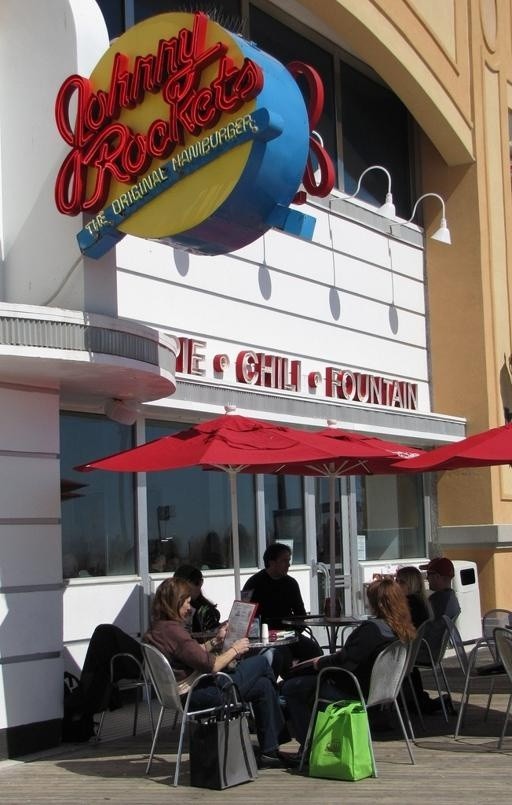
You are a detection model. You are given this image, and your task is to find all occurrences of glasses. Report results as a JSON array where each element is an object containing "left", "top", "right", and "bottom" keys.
[{"left": 427, "top": 571, "right": 439, "bottom": 575}]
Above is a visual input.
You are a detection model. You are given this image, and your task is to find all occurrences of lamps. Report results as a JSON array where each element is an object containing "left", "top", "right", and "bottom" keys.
[{"left": 328, "top": 163, "right": 453, "bottom": 248}]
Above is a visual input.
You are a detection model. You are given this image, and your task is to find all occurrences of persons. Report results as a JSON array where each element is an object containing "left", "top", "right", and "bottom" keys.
[
  {"left": 240, "top": 542, "right": 321, "bottom": 681},
  {"left": 282, "top": 577, "right": 419, "bottom": 765},
  {"left": 393, "top": 566, "right": 435, "bottom": 630},
  {"left": 171, "top": 563, "right": 285, "bottom": 736},
  {"left": 398, "top": 556, "right": 462, "bottom": 714},
  {"left": 141, "top": 577, "right": 300, "bottom": 770}
]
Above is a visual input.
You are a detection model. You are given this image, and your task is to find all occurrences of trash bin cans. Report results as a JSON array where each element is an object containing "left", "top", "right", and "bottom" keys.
[{"left": 418, "top": 560, "right": 483, "bottom": 644}]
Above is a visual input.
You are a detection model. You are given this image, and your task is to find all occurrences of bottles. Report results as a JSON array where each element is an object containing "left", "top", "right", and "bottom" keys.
[{"left": 261, "top": 623, "right": 269, "bottom": 640}]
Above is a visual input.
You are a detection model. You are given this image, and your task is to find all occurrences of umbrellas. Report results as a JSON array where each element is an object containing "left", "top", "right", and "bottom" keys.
[
  {"left": 69, "top": 402, "right": 406, "bottom": 605},
  {"left": 196, "top": 416, "right": 449, "bottom": 620},
  {"left": 387, "top": 415, "right": 512, "bottom": 476}
]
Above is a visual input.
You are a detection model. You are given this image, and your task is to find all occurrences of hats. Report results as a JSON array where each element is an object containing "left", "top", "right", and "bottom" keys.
[{"left": 419, "top": 558, "right": 454, "bottom": 577}]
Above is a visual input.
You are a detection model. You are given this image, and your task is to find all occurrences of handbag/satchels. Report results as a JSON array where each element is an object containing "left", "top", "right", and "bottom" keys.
[
  {"left": 188, "top": 682, "right": 258, "bottom": 790},
  {"left": 310, "top": 700, "right": 372, "bottom": 781}
]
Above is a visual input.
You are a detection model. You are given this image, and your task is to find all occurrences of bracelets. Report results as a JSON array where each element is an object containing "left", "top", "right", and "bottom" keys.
[
  {"left": 208, "top": 639, "right": 217, "bottom": 648},
  {"left": 229, "top": 645, "right": 241, "bottom": 661}
]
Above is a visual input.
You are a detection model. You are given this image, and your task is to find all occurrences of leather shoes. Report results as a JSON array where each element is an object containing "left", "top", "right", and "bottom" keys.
[{"left": 260, "top": 755, "right": 301, "bottom": 769}]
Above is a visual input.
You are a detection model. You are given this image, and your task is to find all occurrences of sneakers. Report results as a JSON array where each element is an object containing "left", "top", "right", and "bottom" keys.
[{"left": 289, "top": 744, "right": 311, "bottom": 763}]
